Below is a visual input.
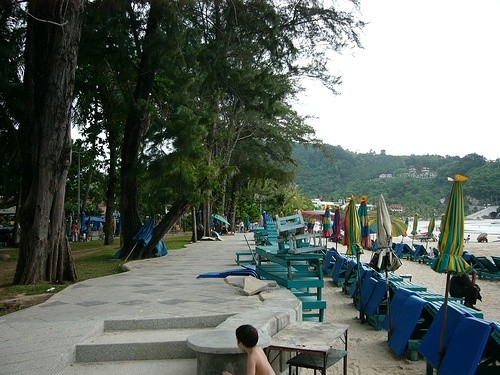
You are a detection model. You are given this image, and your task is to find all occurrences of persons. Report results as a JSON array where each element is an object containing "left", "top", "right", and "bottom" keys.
[
  {"left": 239, "top": 220, "right": 244, "bottom": 233},
  {"left": 221, "top": 227, "right": 229, "bottom": 235},
  {"left": 222, "top": 324, "right": 276, "bottom": 375},
  {"left": 464, "top": 234, "right": 470, "bottom": 240},
  {"left": 449, "top": 269, "right": 483, "bottom": 311},
  {"left": 72, "top": 220, "right": 79, "bottom": 242}
]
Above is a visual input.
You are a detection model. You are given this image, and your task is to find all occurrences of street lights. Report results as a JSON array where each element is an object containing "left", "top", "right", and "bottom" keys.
[{"left": 70, "top": 150, "right": 88, "bottom": 222}]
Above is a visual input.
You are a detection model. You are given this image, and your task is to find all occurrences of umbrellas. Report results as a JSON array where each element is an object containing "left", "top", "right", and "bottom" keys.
[
  {"left": 211, "top": 213, "right": 231, "bottom": 226},
  {"left": 0, "top": 204, "right": 168, "bottom": 259},
  {"left": 305, "top": 174, "right": 470, "bottom": 275}
]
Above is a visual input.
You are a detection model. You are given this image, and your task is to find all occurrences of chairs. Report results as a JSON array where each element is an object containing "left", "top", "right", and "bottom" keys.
[{"left": 323, "top": 239, "right": 500, "bottom": 375}]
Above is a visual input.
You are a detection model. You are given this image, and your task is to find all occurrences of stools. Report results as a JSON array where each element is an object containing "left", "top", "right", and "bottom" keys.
[{"left": 399, "top": 273, "right": 412, "bottom": 282}]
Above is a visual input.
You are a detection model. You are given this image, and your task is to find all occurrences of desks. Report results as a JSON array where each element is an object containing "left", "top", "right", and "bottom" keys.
[
  {"left": 285, "top": 349, "right": 348, "bottom": 375},
  {"left": 264, "top": 320, "right": 349, "bottom": 375}
]
